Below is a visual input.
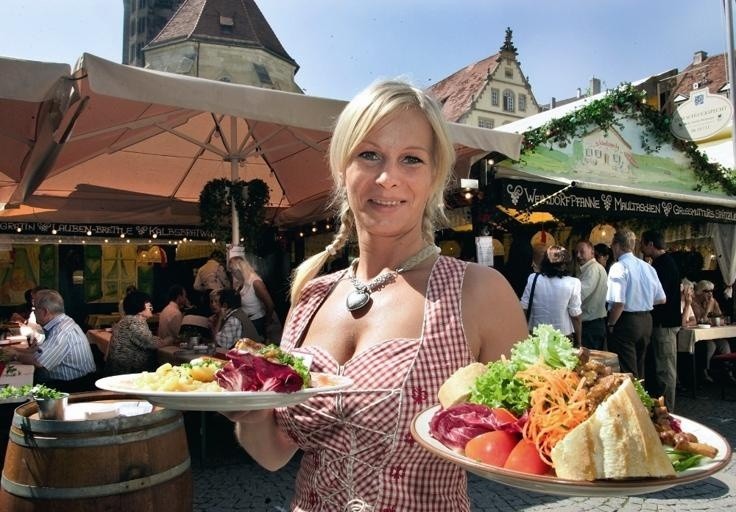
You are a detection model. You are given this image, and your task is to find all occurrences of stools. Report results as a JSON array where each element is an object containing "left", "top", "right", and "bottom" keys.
[{"left": 713, "top": 352, "right": 736, "bottom": 399}]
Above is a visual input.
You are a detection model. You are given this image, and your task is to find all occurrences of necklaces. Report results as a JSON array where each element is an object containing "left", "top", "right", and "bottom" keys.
[{"left": 346, "top": 245, "right": 441, "bottom": 311}]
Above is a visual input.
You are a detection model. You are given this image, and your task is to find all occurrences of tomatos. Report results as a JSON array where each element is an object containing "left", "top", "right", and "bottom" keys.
[
  {"left": 463, "top": 430, "right": 515, "bottom": 466},
  {"left": 503, "top": 438, "right": 548, "bottom": 478}
]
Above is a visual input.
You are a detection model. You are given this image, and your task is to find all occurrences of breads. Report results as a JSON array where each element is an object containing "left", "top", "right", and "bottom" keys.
[
  {"left": 438, "top": 362, "right": 491, "bottom": 410},
  {"left": 550, "top": 375, "right": 677, "bottom": 481}
]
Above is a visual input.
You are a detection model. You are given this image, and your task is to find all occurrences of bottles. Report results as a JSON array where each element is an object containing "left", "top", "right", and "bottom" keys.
[{"left": 698, "top": 312, "right": 732, "bottom": 330}]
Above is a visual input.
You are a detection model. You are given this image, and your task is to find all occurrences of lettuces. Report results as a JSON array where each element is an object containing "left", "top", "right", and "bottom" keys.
[{"left": 472, "top": 323, "right": 651, "bottom": 413}]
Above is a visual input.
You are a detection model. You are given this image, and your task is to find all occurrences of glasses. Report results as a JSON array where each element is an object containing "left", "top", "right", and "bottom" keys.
[{"left": 704, "top": 289, "right": 713, "bottom": 293}]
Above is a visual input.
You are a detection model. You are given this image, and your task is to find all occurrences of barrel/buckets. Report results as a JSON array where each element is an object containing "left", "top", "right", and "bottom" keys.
[
  {"left": 575, "top": 348, "right": 620, "bottom": 375},
  {"left": 0, "top": 390, "right": 194, "bottom": 512}
]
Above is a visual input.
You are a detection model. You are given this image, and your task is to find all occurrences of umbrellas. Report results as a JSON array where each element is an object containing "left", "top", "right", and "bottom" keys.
[
  {"left": 22, "top": 52, "right": 350, "bottom": 247},
  {"left": 0, "top": 57, "right": 72, "bottom": 212}
]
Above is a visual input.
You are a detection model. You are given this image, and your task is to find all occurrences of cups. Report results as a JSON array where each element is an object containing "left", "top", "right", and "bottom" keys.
[{"left": 179, "top": 334, "right": 218, "bottom": 357}]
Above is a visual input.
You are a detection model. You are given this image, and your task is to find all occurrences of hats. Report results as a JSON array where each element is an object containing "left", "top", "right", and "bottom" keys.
[{"left": 547, "top": 245, "right": 569, "bottom": 263}]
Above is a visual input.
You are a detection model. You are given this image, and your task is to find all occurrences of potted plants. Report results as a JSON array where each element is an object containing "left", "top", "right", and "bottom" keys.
[{"left": 0, "top": 382, "right": 69, "bottom": 420}]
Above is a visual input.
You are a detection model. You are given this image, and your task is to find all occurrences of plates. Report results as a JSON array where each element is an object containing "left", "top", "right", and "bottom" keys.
[
  {"left": 408, "top": 401, "right": 731, "bottom": 499},
  {"left": 94, "top": 366, "right": 354, "bottom": 414}
]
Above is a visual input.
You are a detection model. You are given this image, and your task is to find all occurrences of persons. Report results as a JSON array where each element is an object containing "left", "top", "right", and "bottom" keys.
[
  {"left": 219, "top": 79, "right": 530, "bottom": 512},
  {"left": 520, "top": 229, "right": 732, "bottom": 414},
  {"left": 2, "top": 286, "right": 98, "bottom": 393},
  {"left": 105, "top": 252, "right": 273, "bottom": 377}
]
